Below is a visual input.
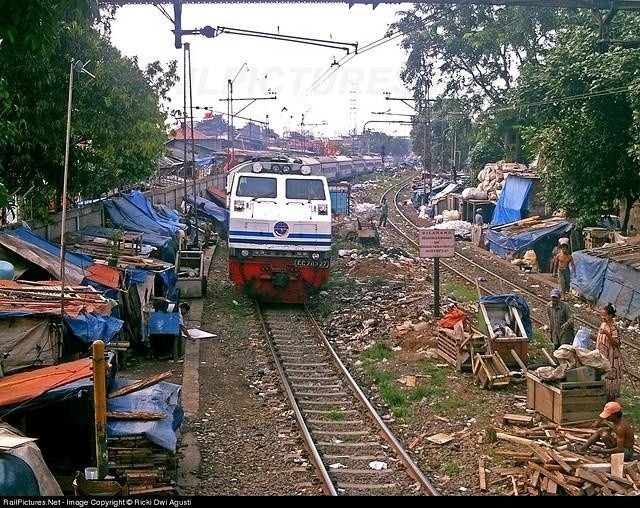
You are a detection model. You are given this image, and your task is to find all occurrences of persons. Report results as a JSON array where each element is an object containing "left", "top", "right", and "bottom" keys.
[
  {"left": 376, "top": 198, "right": 389, "bottom": 227},
  {"left": 204, "top": 219, "right": 212, "bottom": 248},
  {"left": 475, "top": 208, "right": 484, "bottom": 225},
  {"left": 567, "top": 401, "right": 635, "bottom": 462},
  {"left": 152, "top": 298, "right": 196, "bottom": 342},
  {"left": 545, "top": 288, "right": 574, "bottom": 350},
  {"left": 553, "top": 243, "right": 577, "bottom": 301},
  {"left": 589, "top": 303, "right": 620, "bottom": 401}
]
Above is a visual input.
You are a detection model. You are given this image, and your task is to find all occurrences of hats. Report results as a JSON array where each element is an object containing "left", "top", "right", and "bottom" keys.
[
  {"left": 599, "top": 402, "right": 621, "bottom": 419},
  {"left": 550, "top": 289, "right": 560, "bottom": 298},
  {"left": 477, "top": 209, "right": 483, "bottom": 214},
  {"left": 558, "top": 238, "right": 569, "bottom": 245}
]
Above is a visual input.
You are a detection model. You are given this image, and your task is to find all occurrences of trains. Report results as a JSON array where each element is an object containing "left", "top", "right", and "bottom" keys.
[{"left": 225, "top": 152, "right": 397, "bottom": 309}]
